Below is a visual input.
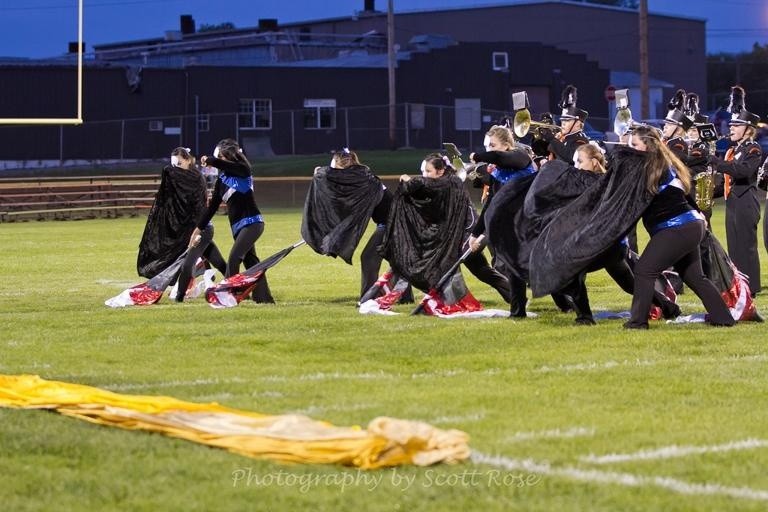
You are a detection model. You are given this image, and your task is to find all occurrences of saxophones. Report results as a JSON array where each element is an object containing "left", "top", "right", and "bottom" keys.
[{"left": 693, "top": 133, "right": 736, "bottom": 210}]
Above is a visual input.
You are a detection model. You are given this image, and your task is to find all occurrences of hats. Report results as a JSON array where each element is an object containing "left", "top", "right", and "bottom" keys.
[
  {"left": 662, "top": 108, "right": 760, "bottom": 133},
  {"left": 559, "top": 106, "right": 588, "bottom": 123}
]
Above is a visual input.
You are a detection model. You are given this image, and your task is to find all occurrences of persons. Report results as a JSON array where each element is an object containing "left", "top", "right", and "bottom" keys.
[
  {"left": 162, "top": 138, "right": 274, "bottom": 303},
  {"left": 314, "top": 107, "right": 768, "bottom": 329}
]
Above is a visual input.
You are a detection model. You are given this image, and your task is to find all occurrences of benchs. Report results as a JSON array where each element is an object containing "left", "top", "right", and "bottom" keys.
[{"left": 0, "top": 175, "right": 228, "bottom": 222}]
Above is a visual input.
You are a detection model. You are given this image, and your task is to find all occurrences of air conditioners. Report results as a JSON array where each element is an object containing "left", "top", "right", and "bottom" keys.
[{"left": 149, "top": 120, "right": 163, "bottom": 132}]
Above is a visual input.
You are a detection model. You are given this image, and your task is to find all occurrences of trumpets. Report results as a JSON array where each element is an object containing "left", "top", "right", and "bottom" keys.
[
  {"left": 453, "top": 158, "right": 487, "bottom": 183},
  {"left": 514, "top": 109, "right": 563, "bottom": 139},
  {"left": 614, "top": 107, "right": 663, "bottom": 140}
]
